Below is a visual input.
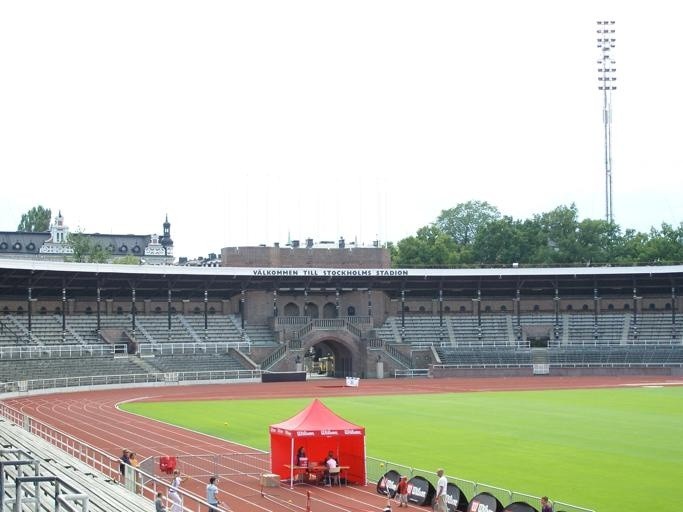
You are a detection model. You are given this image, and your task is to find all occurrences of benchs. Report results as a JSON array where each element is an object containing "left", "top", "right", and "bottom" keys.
[{"left": 0, "top": 419, "right": 187, "bottom": 512}]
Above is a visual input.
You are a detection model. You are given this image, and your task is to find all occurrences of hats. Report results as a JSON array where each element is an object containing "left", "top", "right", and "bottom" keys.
[{"left": 399, "top": 476, "right": 407, "bottom": 480}]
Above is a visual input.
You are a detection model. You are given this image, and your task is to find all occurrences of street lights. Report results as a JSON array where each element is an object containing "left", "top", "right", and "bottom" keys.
[{"left": 596, "top": 19, "right": 618, "bottom": 231}]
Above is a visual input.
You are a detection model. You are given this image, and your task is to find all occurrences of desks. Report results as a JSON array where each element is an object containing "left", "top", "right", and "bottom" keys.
[{"left": 283, "top": 463, "right": 350, "bottom": 486}]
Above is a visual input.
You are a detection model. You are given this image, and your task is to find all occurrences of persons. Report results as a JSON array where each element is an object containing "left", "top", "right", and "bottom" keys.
[
  {"left": 435, "top": 468, "right": 447, "bottom": 512},
  {"left": 540, "top": 495, "right": 551, "bottom": 511},
  {"left": 297, "top": 446, "right": 308, "bottom": 467},
  {"left": 323, "top": 452, "right": 338, "bottom": 482},
  {"left": 118, "top": 449, "right": 138, "bottom": 478},
  {"left": 167, "top": 469, "right": 191, "bottom": 512},
  {"left": 396, "top": 475, "right": 407, "bottom": 508},
  {"left": 206, "top": 476, "right": 221, "bottom": 512},
  {"left": 154, "top": 491, "right": 166, "bottom": 511}
]
